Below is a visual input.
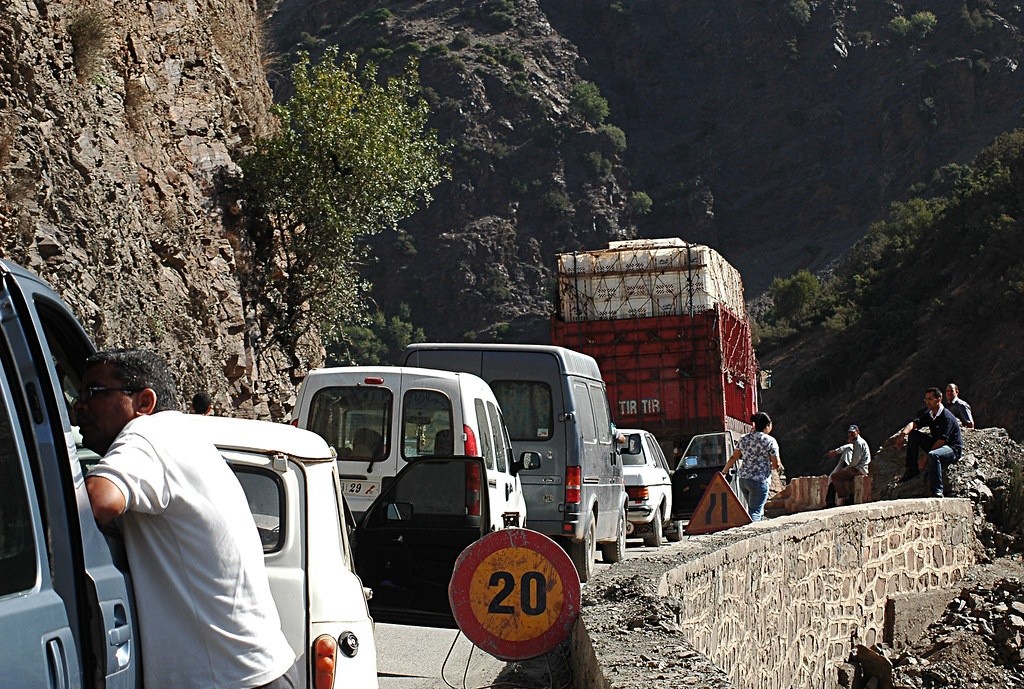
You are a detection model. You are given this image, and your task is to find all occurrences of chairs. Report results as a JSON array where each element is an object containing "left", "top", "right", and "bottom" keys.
[
  {"left": 620, "top": 447, "right": 630, "bottom": 454},
  {"left": 433, "top": 429, "right": 453, "bottom": 456},
  {"left": 353, "top": 428, "right": 384, "bottom": 458}
]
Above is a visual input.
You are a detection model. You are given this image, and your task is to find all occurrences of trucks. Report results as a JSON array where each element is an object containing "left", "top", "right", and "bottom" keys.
[{"left": 551, "top": 301, "right": 771, "bottom": 518}]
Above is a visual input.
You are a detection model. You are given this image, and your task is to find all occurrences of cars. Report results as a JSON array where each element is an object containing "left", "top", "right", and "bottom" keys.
[
  {"left": 184, "top": 417, "right": 493, "bottom": 689},
  {"left": 614, "top": 429, "right": 742, "bottom": 550}
]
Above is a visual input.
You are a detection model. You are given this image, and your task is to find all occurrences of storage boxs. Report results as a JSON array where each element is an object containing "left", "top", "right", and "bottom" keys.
[{"left": 555, "top": 237, "right": 744, "bottom": 323}]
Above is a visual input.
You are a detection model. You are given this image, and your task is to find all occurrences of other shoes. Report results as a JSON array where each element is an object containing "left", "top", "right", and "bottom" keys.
[{"left": 898, "top": 468, "right": 920, "bottom": 483}]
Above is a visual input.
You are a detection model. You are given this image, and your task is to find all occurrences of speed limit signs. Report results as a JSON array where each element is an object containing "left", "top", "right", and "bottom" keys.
[{"left": 448, "top": 528, "right": 582, "bottom": 663}]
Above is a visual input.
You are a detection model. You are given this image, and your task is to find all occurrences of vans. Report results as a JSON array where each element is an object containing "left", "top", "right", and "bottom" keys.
[
  {"left": 400, "top": 343, "right": 641, "bottom": 585},
  {"left": 290, "top": 366, "right": 543, "bottom": 535},
  {"left": 0, "top": 259, "right": 143, "bottom": 689}
]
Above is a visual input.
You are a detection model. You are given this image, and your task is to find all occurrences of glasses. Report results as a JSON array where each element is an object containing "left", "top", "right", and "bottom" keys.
[
  {"left": 946, "top": 390, "right": 954, "bottom": 392},
  {"left": 924, "top": 398, "right": 937, "bottom": 401},
  {"left": 77, "top": 384, "right": 159, "bottom": 403}
]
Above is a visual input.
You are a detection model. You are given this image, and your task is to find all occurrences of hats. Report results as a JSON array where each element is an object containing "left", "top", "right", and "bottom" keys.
[{"left": 848, "top": 425, "right": 859, "bottom": 432}]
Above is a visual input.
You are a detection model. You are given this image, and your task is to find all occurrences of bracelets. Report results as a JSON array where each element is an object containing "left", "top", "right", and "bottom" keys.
[
  {"left": 900, "top": 433, "right": 906, "bottom": 435},
  {"left": 722, "top": 470, "right": 727, "bottom": 474}
]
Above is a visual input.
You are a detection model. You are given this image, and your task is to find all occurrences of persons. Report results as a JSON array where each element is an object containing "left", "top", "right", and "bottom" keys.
[
  {"left": 611, "top": 424, "right": 626, "bottom": 444},
  {"left": 944, "top": 383, "right": 974, "bottom": 427},
  {"left": 895, "top": 387, "right": 962, "bottom": 497},
  {"left": 77, "top": 348, "right": 299, "bottom": 689},
  {"left": 821, "top": 425, "right": 871, "bottom": 509},
  {"left": 192, "top": 393, "right": 211, "bottom": 416},
  {"left": 723, "top": 412, "right": 785, "bottom": 522}
]
